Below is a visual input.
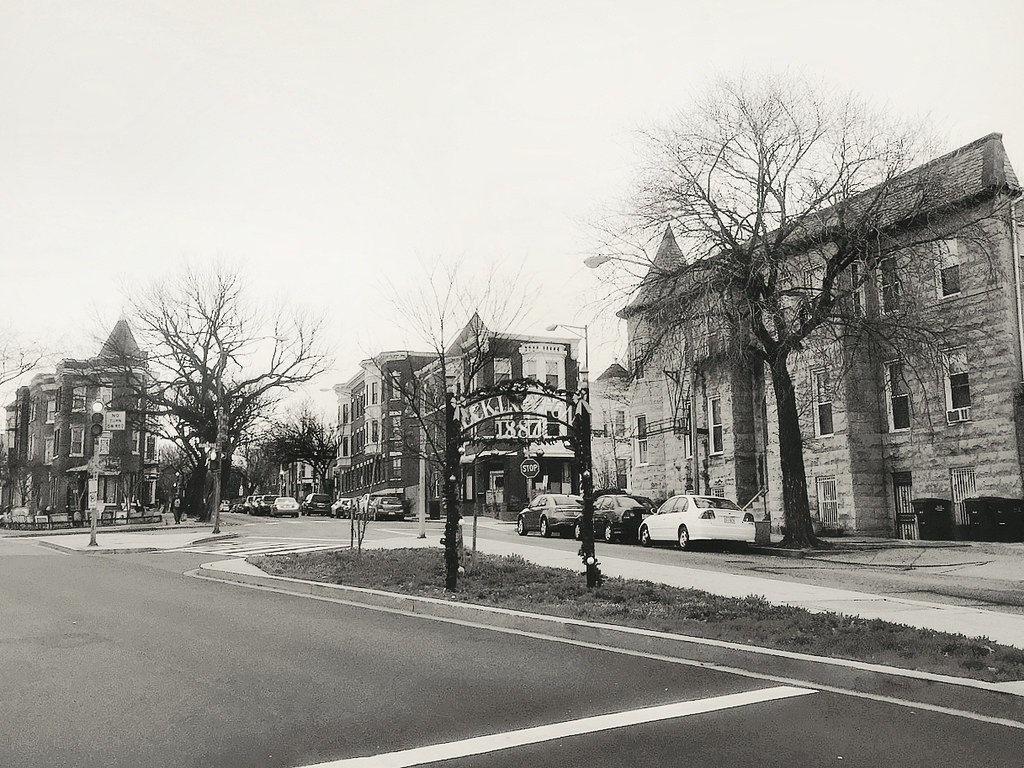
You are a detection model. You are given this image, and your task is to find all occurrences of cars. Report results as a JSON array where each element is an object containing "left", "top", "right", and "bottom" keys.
[
  {"left": 637, "top": 495, "right": 757, "bottom": 553},
  {"left": 331, "top": 494, "right": 405, "bottom": 521},
  {"left": 269, "top": 497, "right": 300, "bottom": 517},
  {"left": 220, "top": 493, "right": 282, "bottom": 517},
  {"left": 572, "top": 494, "right": 659, "bottom": 542},
  {"left": 517, "top": 494, "right": 581, "bottom": 538}
]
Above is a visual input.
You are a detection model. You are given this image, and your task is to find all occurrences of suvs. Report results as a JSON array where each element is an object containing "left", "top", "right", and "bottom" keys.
[{"left": 300, "top": 493, "right": 333, "bottom": 518}]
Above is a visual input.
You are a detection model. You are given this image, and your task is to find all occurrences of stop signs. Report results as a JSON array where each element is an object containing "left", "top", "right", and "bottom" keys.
[{"left": 521, "top": 458, "right": 540, "bottom": 478}]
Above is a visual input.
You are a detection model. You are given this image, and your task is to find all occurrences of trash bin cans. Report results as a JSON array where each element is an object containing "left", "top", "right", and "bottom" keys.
[
  {"left": 963, "top": 496, "right": 1023, "bottom": 543},
  {"left": 910, "top": 498, "right": 951, "bottom": 541},
  {"left": 428, "top": 500, "right": 441, "bottom": 520}
]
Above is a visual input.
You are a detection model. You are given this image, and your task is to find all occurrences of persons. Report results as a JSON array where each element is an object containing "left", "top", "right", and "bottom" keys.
[{"left": 170, "top": 493, "right": 185, "bottom": 524}]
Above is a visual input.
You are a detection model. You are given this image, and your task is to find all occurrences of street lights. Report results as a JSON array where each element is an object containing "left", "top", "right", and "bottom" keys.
[{"left": 582, "top": 255, "right": 701, "bottom": 494}]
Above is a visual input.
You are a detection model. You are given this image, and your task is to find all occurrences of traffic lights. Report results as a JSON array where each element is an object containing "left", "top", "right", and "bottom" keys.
[
  {"left": 208, "top": 449, "right": 219, "bottom": 472},
  {"left": 89, "top": 399, "right": 105, "bottom": 438}
]
